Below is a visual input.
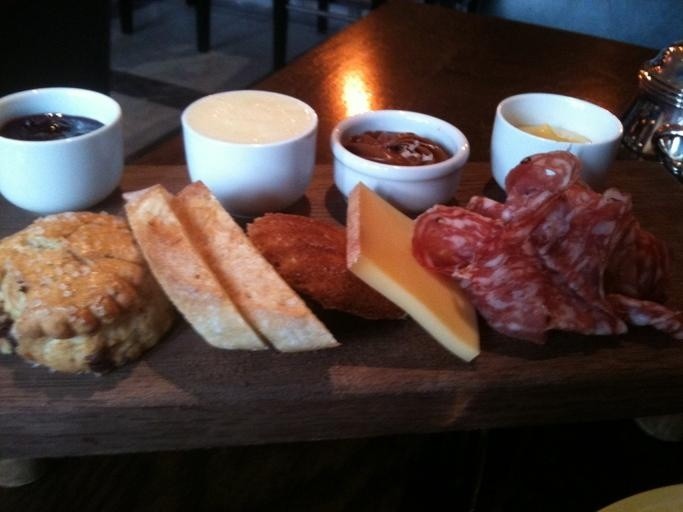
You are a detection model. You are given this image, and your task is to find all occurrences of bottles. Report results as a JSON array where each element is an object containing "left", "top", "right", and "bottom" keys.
[{"left": 622, "top": 45, "right": 682, "bottom": 161}]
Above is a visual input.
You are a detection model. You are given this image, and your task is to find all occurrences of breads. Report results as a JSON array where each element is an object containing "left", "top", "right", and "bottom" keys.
[
  {"left": 122, "top": 179, "right": 341, "bottom": 355},
  {"left": 0, "top": 208, "right": 178, "bottom": 376}
]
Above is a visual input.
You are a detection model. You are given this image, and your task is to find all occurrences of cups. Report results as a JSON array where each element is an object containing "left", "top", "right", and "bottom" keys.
[
  {"left": 491, "top": 92, "right": 626, "bottom": 196},
  {"left": 182, "top": 90, "right": 319, "bottom": 220},
  {"left": 1, "top": 87, "right": 124, "bottom": 217},
  {"left": 331, "top": 110, "right": 471, "bottom": 214}
]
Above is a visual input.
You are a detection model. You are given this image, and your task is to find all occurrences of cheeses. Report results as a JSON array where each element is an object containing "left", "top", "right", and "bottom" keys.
[{"left": 347, "top": 181, "right": 485, "bottom": 364}]
